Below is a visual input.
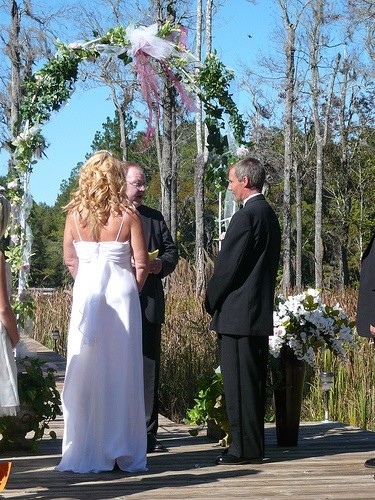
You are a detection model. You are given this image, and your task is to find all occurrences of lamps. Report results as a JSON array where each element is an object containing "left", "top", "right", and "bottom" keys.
[
  {"left": 51, "top": 326, "right": 59, "bottom": 340},
  {"left": 320, "top": 372, "right": 335, "bottom": 392}
]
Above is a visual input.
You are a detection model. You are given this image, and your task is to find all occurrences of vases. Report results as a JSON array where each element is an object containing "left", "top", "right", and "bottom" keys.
[{"left": 271, "top": 345, "right": 306, "bottom": 448}]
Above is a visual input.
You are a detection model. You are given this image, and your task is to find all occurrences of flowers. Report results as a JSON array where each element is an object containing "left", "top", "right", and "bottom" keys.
[
  {"left": 3, "top": 22, "right": 252, "bottom": 337},
  {"left": 270, "top": 290, "right": 357, "bottom": 369},
  {"left": 219, "top": 231, "right": 226, "bottom": 241}
]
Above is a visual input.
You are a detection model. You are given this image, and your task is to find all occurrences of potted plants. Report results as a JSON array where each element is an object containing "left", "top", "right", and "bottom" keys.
[
  {"left": 0, "top": 357, "right": 62, "bottom": 450},
  {"left": 179, "top": 366, "right": 230, "bottom": 445}
]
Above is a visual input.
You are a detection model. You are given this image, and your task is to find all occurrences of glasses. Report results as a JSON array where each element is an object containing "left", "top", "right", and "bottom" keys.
[{"left": 126, "top": 180, "right": 150, "bottom": 192}]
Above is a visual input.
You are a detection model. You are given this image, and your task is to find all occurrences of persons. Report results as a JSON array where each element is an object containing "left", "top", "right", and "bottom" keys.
[
  {"left": 355, "top": 234, "right": 375, "bottom": 480},
  {"left": 204, "top": 158, "right": 280, "bottom": 465},
  {"left": 122, "top": 159, "right": 178, "bottom": 453},
  {"left": 54, "top": 148, "right": 150, "bottom": 472},
  {"left": 0, "top": 191, "right": 21, "bottom": 416}
]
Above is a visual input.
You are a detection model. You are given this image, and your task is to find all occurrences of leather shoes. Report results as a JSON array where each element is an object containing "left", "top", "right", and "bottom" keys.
[
  {"left": 365, "top": 458, "right": 375, "bottom": 467},
  {"left": 147, "top": 436, "right": 164, "bottom": 451},
  {"left": 222, "top": 447, "right": 229, "bottom": 454},
  {"left": 216, "top": 453, "right": 264, "bottom": 465}
]
